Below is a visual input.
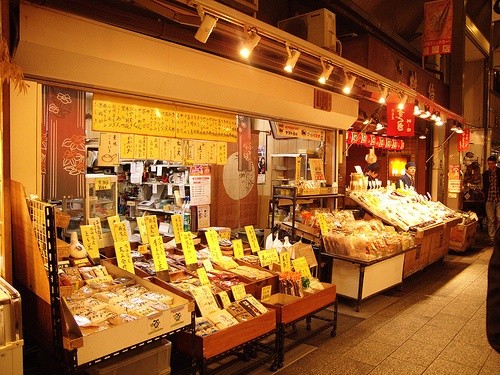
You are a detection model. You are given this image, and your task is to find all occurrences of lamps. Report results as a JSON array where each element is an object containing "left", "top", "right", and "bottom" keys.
[
  {"left": 376, "top": 80, "right": 389, "bottom": 104},
  {"left": 318, "top": 56, "right": 334, "bottom": 84},
  {"left": 396, "top": 90, "right": 408, "bottom": 110},
  {"left": 463, "top": 152, "right": 474, "bottom": 163},
  {"left": 194, "top": 5, "right": 218, "bottom": 44},
  {"left": 372, "top": 116, "right": 384, "bottom": 130},
  {"left": 343, "top": 67, "right": 357, "bottom": 94},
  {"left": 413, "top": 99, "right": 443, "bottom": 139},
  {"left": 450, "top": 119, "right": 463, "bottom": 133},
  {"left": 284, "top": 40, "right": 300, "bottom": 72},
  {"left": 359, "top": 108, "right": 370, "bottom": 125},
  {"left": 239, "top": 23, "right": 261, "bottom": 53}
]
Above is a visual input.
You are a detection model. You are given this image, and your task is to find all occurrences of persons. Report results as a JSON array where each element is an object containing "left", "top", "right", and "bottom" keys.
[
  {"left": 364, "top": 163, "right": 380, "bottom": 189},
  {"left": 459, "top": 152, "right": 500, "bottom": 246},
  {"left": 403, "top": 162, "right": 417, "bottom": 188}
]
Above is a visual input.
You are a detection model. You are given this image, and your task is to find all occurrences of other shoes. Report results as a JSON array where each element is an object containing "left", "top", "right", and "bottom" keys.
[{"left": 489, "top": 241, "right": 494, "bottom": 245}]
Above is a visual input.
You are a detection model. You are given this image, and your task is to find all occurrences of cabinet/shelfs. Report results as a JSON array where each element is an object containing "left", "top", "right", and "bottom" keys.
[
  {"left": 270, "top": 154, "right": 316, "bottom": 213},
  {"left": 272, "top": 185, "right": 338, "bottom": 243},
  {"left": 63, "top": 174, "right": 118, "bottom": 243},
  {"left": 134, "top": 165, "right": 196, "bottom": 235}
]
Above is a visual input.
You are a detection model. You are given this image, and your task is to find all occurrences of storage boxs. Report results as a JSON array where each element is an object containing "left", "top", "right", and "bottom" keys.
[{"left": 55, "top": 184, "right": 350, "bottom": 375}]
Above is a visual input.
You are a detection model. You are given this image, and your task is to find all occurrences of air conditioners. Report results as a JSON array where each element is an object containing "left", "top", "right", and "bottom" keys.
[
  {"left": 221, "top": 0, "right": 259, "bottom": 15},
  {"left": 277, "top": 8, "right": 336, "bottom": 53}
]
